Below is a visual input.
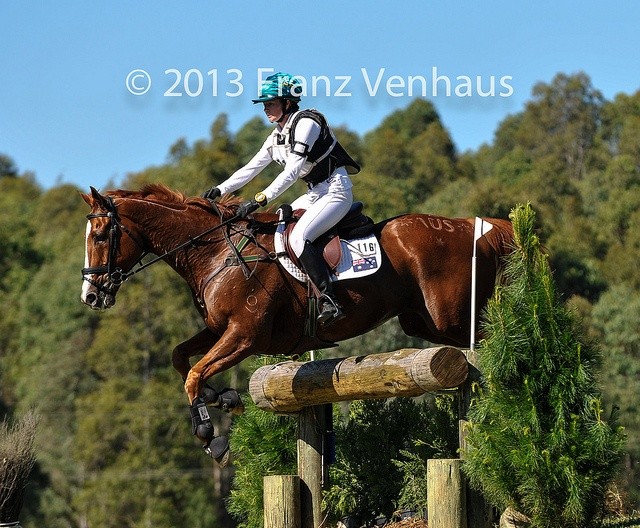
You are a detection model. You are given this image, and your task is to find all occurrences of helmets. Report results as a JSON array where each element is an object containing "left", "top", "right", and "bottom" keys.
[{"left": 252, "top": 72, "right": 302, "bottom": 104}]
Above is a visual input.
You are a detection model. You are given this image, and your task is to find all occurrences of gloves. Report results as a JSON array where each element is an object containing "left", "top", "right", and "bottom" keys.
[
  {"left": 236, "top": 200, "right": 260, "bottom": 220},
  {"left": 200, "top": 188, "right": 221, "bottom": 200}
]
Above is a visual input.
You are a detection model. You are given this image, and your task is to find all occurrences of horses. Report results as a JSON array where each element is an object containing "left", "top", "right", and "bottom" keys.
[{"left": 77, "top": 182, "right": 552, "bottom": 470}]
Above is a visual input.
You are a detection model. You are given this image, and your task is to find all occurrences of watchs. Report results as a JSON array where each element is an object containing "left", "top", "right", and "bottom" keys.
[{"left": 253, "top": 192, "right": 267, "bottom": 208}]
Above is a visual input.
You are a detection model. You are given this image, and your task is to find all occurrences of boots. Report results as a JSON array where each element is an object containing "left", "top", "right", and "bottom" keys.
[{"left": 297, "top": 239, "right": 346, "bottom": 324}]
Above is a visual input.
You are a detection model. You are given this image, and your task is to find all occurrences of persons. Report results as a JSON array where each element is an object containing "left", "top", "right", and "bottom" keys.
[{"left": 203, "top": 72, "right": 360, "bottom": 324}]
